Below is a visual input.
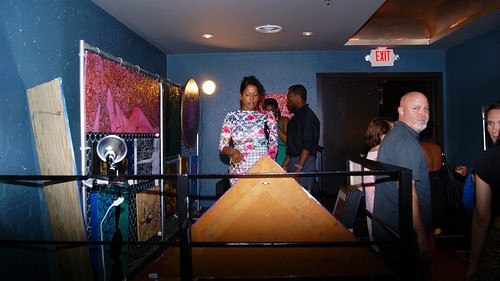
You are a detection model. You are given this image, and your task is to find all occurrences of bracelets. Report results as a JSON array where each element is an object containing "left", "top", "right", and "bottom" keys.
[{"left": 294, "top": 164, "right": 303, "bottom": 169}]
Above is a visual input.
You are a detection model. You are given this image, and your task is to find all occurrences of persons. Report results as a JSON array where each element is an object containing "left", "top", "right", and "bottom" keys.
[
  {"left": 416, "top": 115, "right": 450, "bottom": 281},
  {"left": 454, "top": 166, "right": 468, "bottom": 177},
  {"left": 470, "top": 103, "right": 500, "bottom": 184},
  {"left": 262, "top": 96, "right": 291, "bottom": 172},
  {"left": 218, "top": 75, "right": 280, "bottom": 186},
  {"left": 464, "top": 130, "right": 500, "bottom": 281},
  {"left": 370, "top": 90, "right": 439, "bottom": 281},
  {"left": 280, "top": 84, "right": 321, "bottom": 195},
  {"left": 363, "top": 117, "right": 395, "bottom": 243}
]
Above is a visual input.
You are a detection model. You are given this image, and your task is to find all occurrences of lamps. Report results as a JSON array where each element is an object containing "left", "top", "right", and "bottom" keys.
[{"left": 96, "top": 134, "right": 128, "bottom": 181}]
